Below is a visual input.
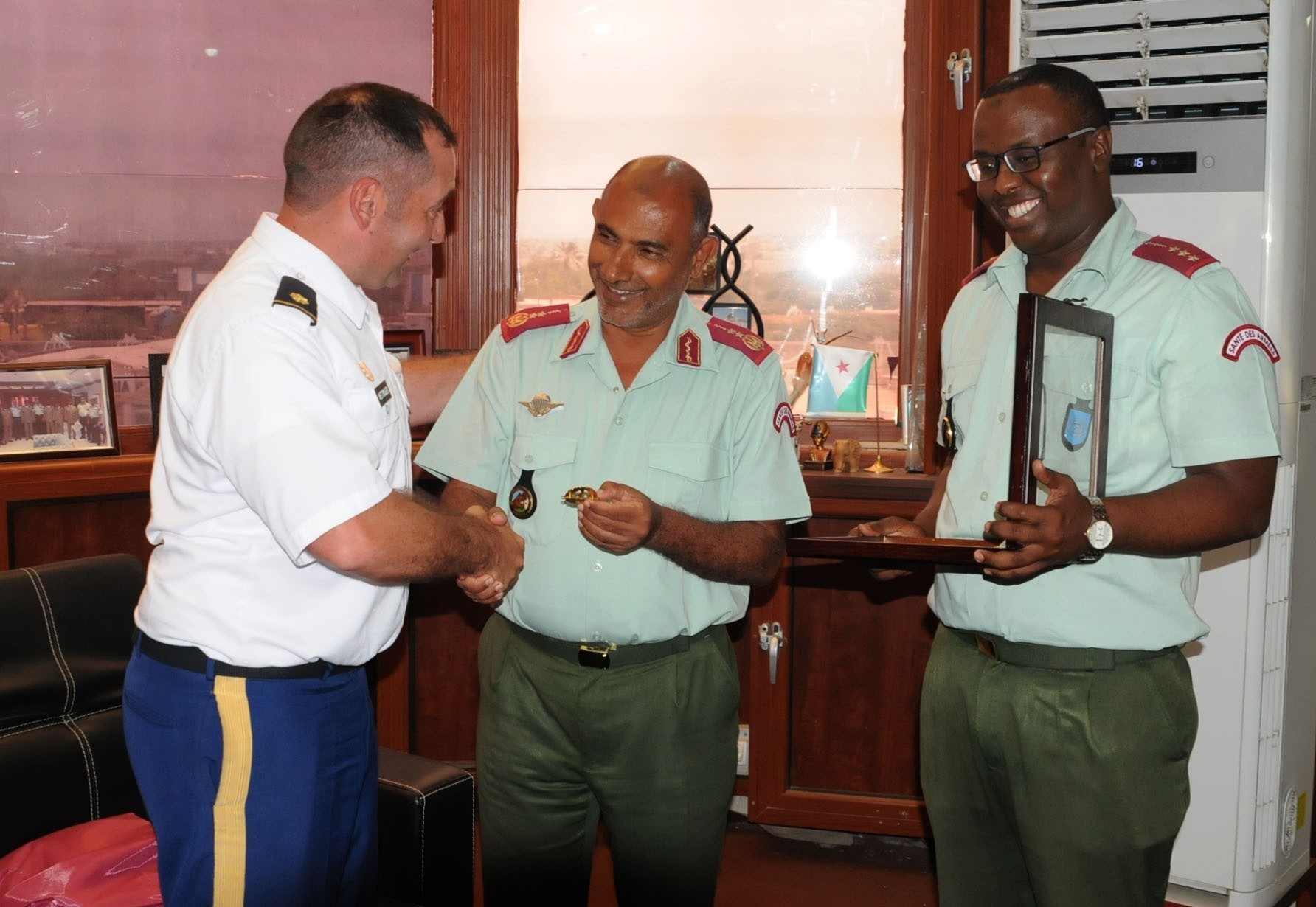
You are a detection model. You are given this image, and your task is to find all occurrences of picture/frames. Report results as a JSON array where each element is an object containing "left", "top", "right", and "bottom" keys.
[
  {"left": 0, "top": 359, "right": 122, "bottom": 462},
  {"left": 148, "top": 329, "right": 431, "bottom": 454}
]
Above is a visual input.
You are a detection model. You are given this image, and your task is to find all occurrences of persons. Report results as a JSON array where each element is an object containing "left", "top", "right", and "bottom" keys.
[
  {"left": 413, "top": 155, "right": 812, "bottom": 906},
  {"left": 124, "top": 82, "right": 524, "bottom": 907},
  {"left": 845, "top": 65, "right": 1279, "bottom": 907},
  {"left": 1, "top": 393, "right": 107, "bottom": 446}
]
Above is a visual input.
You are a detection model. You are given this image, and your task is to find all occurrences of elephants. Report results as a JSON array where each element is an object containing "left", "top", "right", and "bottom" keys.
[{"left": 831, "top": 438, "right": 862, "bottom": 473}]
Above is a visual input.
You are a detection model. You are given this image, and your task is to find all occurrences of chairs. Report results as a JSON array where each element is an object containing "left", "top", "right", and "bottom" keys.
[{"left": 0, "top": 552, "right": 151, "bottom": 857}]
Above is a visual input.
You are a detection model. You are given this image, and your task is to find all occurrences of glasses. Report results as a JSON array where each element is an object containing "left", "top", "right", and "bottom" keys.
[{"left": 962, "top": 127, "right": 1097, "bottom": 183}]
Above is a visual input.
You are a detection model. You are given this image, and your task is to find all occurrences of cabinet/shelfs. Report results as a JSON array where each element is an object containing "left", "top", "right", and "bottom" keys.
[{"left": 1, "top": 496, "right": 938, "bottom": 837}]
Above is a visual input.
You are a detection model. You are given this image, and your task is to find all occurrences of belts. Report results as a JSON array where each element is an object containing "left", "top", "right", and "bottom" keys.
[
  {"left": 129, "top": 625, "right": 368, "bottom": 681},
  {"left": 507, "top": 621, "right": 719, "bottom": 670},
  {"left": 950, "top": 626, "right": 1182, "bottom": 671}
]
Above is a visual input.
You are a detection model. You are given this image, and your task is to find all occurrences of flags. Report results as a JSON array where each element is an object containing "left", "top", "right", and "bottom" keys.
[{"left": 806, "top": 345, "right": 874, "bottom": 414}]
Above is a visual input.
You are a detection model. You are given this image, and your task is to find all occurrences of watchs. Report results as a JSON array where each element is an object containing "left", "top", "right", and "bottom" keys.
[{"left": 1079, "top": 495, "right": 1113, "bottom": 563}]
